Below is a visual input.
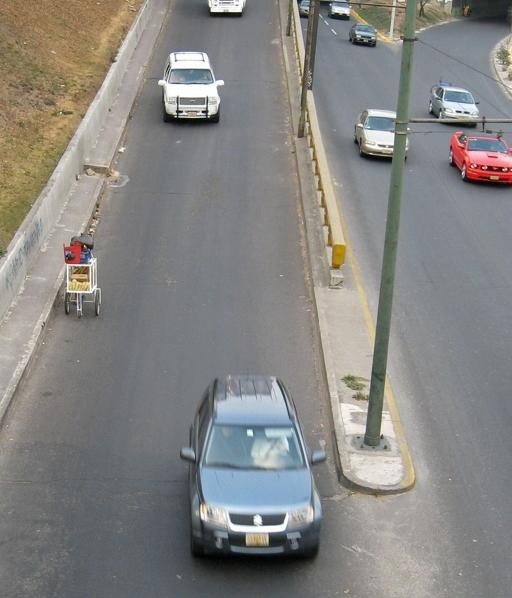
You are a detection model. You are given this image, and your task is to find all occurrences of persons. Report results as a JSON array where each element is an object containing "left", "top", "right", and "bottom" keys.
[
  {"left": 207, "top": 426, "right": 247, "bottom": 466},
  {"left": 251, "top": 433, "right": 289, "bottom": 469}
]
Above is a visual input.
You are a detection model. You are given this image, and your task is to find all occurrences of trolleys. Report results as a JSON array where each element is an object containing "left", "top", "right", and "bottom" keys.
[{"left": 64, "top": 233, "right": 101, "bottom": 319}]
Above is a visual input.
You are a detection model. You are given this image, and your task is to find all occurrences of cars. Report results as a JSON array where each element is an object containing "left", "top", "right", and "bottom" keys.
[
  {"left": 349, "top": 22, "right": 377, "bottom": 47},
  {"left": 353, "top": 109, "right": 410, "bottom": 160},
  {"left": 328, "top": 0, "right": 352, "bottom": 20},
  {"left": 208, "top": 0, "right": 246, "bottom": 17},
  {"left": 427, "top": 85, "right": 512, "bottom": 186},
  {"left": 157, "top": 51, "right": 224, "bottom": 122},
  {"left": 299, "top": 0, "right": 310, "bottom": 18},
  {"left": 180, "top": 373, "right": 326, "bottom": 558}
]
